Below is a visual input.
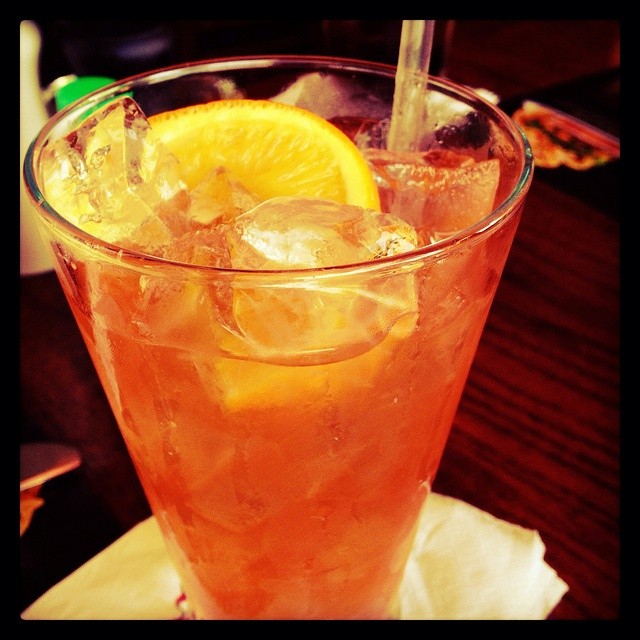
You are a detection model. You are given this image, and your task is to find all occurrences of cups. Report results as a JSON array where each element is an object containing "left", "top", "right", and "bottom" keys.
[{"left": 22, "top": 56, "right": 536, "bottom": 620}]
